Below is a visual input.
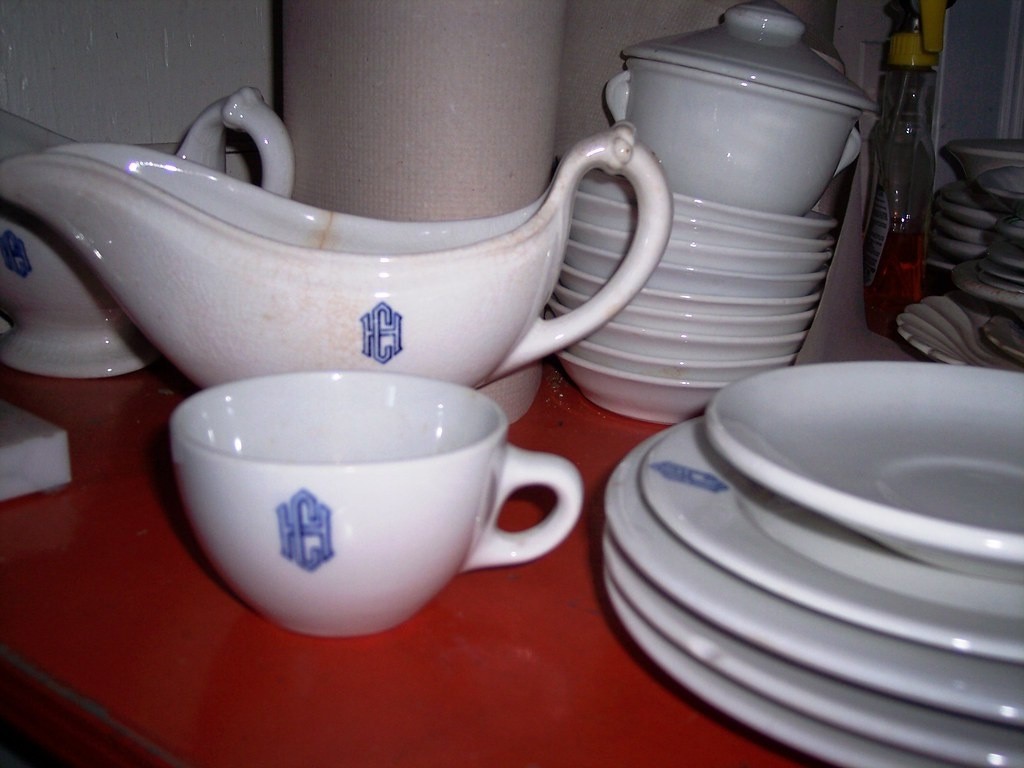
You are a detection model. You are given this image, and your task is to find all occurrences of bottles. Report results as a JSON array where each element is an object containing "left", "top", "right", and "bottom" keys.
[{"left": 862, "top": 34, "right": 939, "bottom": 337}]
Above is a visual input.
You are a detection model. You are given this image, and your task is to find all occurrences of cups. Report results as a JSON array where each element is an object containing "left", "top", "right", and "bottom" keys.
[{"left": 168, "top": 370, "right": 583, "bottom": 637}]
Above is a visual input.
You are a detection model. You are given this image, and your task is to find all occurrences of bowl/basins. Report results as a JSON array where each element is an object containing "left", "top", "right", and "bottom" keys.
[{"left": 556, "top": 0, "right": 881, "bottom": 425}]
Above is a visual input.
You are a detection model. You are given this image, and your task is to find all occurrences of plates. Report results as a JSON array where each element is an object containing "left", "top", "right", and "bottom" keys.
[
  {"left": 602, "top": 359, "right": 1024, "bottom": 768},
  {"left": 897, "top": 137, "right": 1024, "bottom": 370}
]
